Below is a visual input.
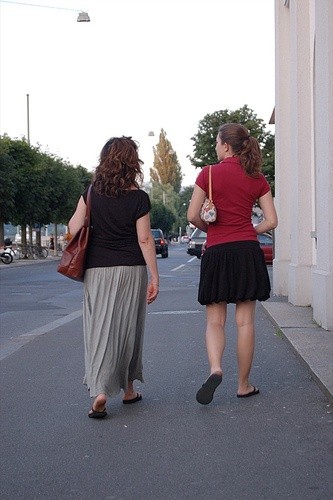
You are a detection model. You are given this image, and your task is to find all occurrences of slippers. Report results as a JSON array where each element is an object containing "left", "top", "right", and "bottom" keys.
[
  {"left": 123, "top": 393, "right": 142, "bottom": 403},
  {"left": 237, "top": 385, "right": 259, "bottom": 398},
  {"left": 196, "top": 374, "right": 222, "bottom": 405},
  {"left": 88, "top": 408, "right": 107, "bottom": 417}
]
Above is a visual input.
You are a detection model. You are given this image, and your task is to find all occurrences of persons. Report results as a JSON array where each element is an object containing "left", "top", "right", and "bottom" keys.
[
  {"left": 187, "top": 124, "right": 279, "bottom": 405},
  {"left": 69, "top": 135, "right": 160, "bottom": 419},
  {"left": 50, "top": 233, "right": 64, "bottom": 250}
]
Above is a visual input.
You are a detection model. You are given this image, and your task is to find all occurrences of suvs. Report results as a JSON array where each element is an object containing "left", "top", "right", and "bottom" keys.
[
  {"left": 186, "top": 225, "right": 207, "bottom": 259},
  {"left": 149, "top": 229, "right": 168, "bottom": 258}
]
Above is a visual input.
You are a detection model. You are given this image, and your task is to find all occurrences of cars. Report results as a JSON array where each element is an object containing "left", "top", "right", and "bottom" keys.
[{"left": 257, "top": 232, "right": 273, "bottom": 263}]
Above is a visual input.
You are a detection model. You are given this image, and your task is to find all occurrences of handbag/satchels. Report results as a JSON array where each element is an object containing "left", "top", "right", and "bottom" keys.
[
  {"left": 58, "top": 184, "right": 91, "bottom": 282},
  {"left": 200, "top": 165, "right": 216, "bottom": 222}
]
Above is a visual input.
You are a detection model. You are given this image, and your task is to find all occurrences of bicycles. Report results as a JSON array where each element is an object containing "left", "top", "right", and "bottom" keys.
[
  {"left": 17, "top": 243, "right": 49, "bottom": 260},
  {"left": 0, "top": 252, "right": 13, "bottom": 265}
]
